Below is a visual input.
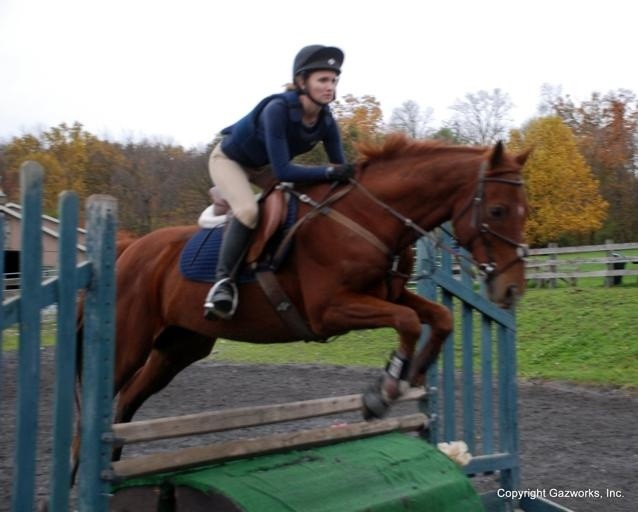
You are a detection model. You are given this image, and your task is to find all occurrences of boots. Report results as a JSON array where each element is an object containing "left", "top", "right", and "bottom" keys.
[{"left": 204, "top": 216, "right": 255, "bottom": 321}]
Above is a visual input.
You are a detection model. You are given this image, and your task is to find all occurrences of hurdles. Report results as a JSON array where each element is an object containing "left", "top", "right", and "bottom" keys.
[{"left": 0, "top": 160, "right": 573, "bottom": 512}]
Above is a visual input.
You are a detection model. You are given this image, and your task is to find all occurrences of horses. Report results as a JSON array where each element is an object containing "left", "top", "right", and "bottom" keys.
[{"left": 68, "top": 130, "right": 538, "bottom": 489}]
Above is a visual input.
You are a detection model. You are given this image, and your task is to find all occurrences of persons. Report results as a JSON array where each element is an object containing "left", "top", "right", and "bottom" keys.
[{"left": 204, "top": 45, "right": 355, "bottom": 322}]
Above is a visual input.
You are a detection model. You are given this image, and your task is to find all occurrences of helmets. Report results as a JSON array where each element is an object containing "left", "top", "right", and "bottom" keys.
[{"left": 294, "top": 45, "right": 343, "bottom": 74}]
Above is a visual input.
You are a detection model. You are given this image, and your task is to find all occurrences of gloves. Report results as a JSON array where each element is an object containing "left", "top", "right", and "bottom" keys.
[{"left": 326, "top": 166, "right": 353, "bottom": 185}]
